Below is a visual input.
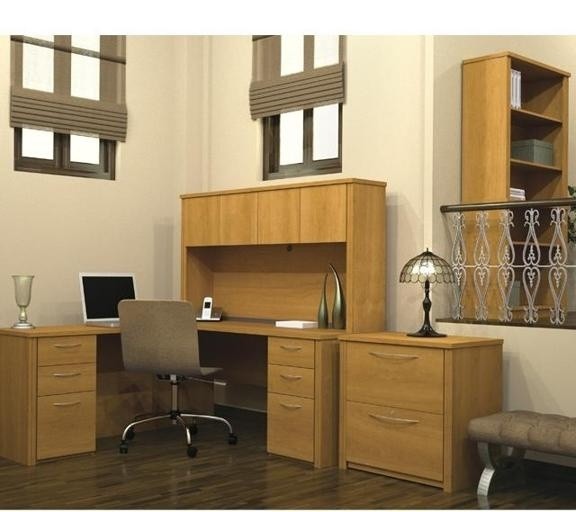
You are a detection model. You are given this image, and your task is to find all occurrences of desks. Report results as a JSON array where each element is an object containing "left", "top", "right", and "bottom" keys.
[{"left": 1, "top": 315, "right": 345, "bottom": 473}]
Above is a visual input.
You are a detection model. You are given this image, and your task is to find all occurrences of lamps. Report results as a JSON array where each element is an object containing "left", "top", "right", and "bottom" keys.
[{"left": 398, "top": 247, "right": 458, "bottom": 337}]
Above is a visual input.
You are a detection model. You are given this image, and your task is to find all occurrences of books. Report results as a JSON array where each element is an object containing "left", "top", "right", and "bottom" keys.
[
  {"left": 510, "top": 188, "right": 526, "bottom": 196},
  {"left": 510, "top": 194, "right": 527, "bottom": 201},
  {"left": 511, "top": 69, "right": 522, "bottom": 109}
]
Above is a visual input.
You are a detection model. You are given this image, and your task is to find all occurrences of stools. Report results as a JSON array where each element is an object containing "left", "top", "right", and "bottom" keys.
[{"left": 465, "top": 409, "right": 576, "bottom": 508}]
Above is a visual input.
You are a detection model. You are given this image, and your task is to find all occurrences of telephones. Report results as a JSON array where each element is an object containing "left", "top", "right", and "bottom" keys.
[{"left": 196, "top": 296, "right": 222, "bottom": 321}]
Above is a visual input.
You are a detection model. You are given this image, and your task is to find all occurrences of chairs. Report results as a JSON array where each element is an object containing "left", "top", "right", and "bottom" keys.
[{"left": 117, "top": 298, "right": 240, "bottom": 459}]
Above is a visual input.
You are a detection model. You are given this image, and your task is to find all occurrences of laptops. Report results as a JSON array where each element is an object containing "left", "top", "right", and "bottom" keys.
[{"left": 79, "top": 272, "right": 137, "bottom": 328}]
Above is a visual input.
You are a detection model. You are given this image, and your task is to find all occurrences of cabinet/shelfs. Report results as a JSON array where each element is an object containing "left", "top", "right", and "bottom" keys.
[
  {"left": 336, "top": 327, "right": 509, "bottom": 495},
  {"left": 462, "top": 50, "right": 573, "bottom": 325},
  {"left": 178, "top": 177, "right": 388, "bottom": 335}
]
